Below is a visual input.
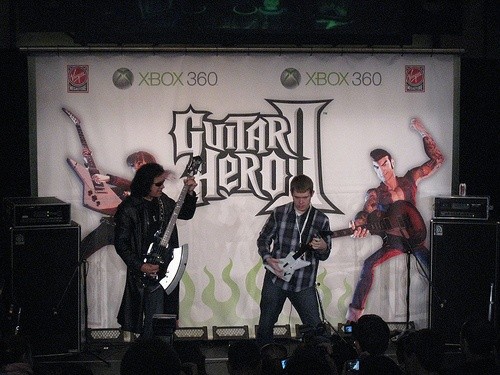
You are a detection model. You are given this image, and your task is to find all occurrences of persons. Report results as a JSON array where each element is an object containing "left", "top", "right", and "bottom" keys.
[
  {"left": 114, "top": 163, "right": 197, "bottom": 342},
  {"left": 0, "top": 315, "right": 499, "bottom": 375},
  {"left": 255, "top": 174, "right": 332, "bottom": 362}
]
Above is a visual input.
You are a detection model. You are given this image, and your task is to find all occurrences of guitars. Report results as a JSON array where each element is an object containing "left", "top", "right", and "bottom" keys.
[
  {"left": 135, "top": 156, "right": 204, "bottom": 292},
  {"left": 262, "top": 229, "right": 334, "bottom": 280}
]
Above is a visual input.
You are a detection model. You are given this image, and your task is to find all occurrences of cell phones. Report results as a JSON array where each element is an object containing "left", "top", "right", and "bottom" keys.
[
  {"left": 344, "top": 325, "right": 352, "bottom": 335},
  {"left": 348, "top": 361, "right": 359, "bottom": 371},
  {"left": 280, "top": 356, "right": 291, "bottom": 371}
]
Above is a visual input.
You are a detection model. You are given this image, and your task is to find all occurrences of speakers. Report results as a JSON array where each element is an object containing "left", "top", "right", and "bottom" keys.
[
  {"left": 428, "top": 220, "right": 499, "bottom": 351},
  {"left": 0, "top": 217, "right": 82, "bottom": 358}
]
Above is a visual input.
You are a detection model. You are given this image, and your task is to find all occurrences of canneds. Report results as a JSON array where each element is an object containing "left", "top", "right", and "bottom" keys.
[{"left": 459, "top": 183, "right": 466, "bottom": 197}]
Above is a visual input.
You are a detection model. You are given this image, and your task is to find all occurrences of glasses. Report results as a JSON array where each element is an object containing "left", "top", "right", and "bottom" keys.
[{"left": 153, "top": 182, "right": 163, "bottom": 186}]
